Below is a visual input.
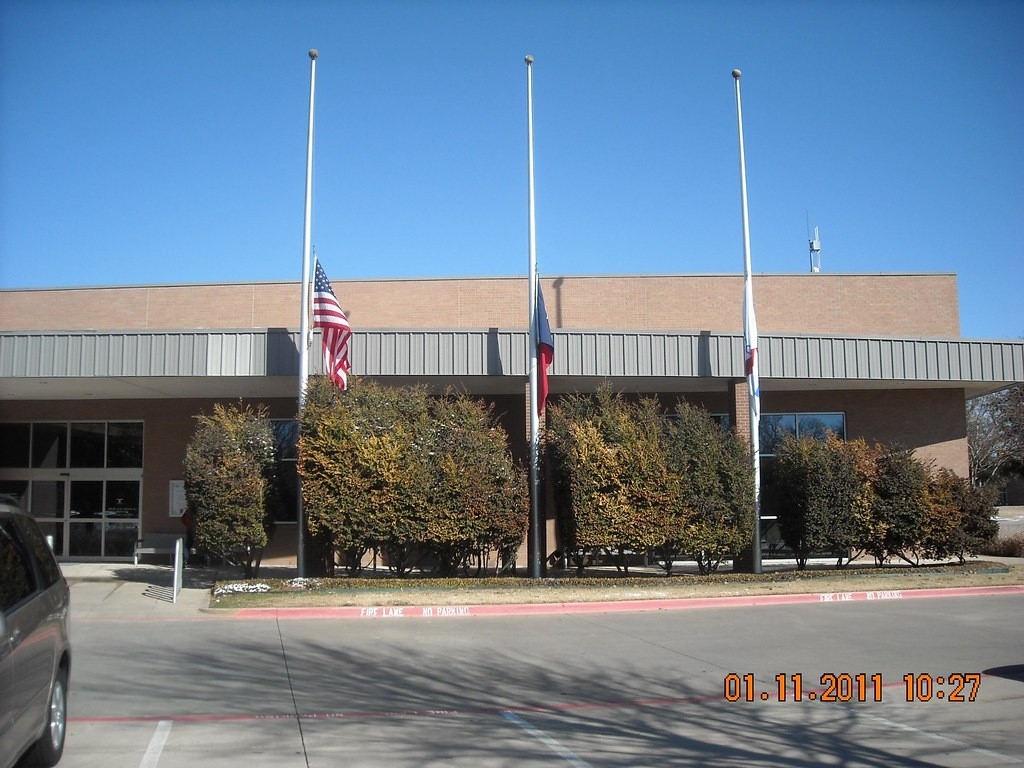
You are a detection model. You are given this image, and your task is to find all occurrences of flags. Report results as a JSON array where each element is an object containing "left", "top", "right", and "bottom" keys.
[
  {"left": 536, "top": 272, "right": 554, "bottom": 417},
  {"left": 743, "top": 278, "right": 760, "bottom": 421},
  {"left": 309, "top": 256, "right": 352, "bottom": 393}
]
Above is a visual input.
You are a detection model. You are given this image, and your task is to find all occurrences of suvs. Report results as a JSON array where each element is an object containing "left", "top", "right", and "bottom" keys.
[{"left": 0, "top": 492, "right": 72, "bottom": 768}]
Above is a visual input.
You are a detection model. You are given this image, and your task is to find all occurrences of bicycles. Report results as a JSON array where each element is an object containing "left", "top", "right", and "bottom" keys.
[{"left": 191, "top": 540, "right": 253, "bottom": 569}]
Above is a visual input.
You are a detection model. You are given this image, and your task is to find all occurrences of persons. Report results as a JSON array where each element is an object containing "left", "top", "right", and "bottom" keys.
[{"left": 180, "top": 507, "right": 212, "bottom": 569}]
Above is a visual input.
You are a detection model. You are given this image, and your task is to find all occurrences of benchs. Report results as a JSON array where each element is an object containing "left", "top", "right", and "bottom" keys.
[{"left": 133, "top": 533, "right": 189, "bottom": 567}]
